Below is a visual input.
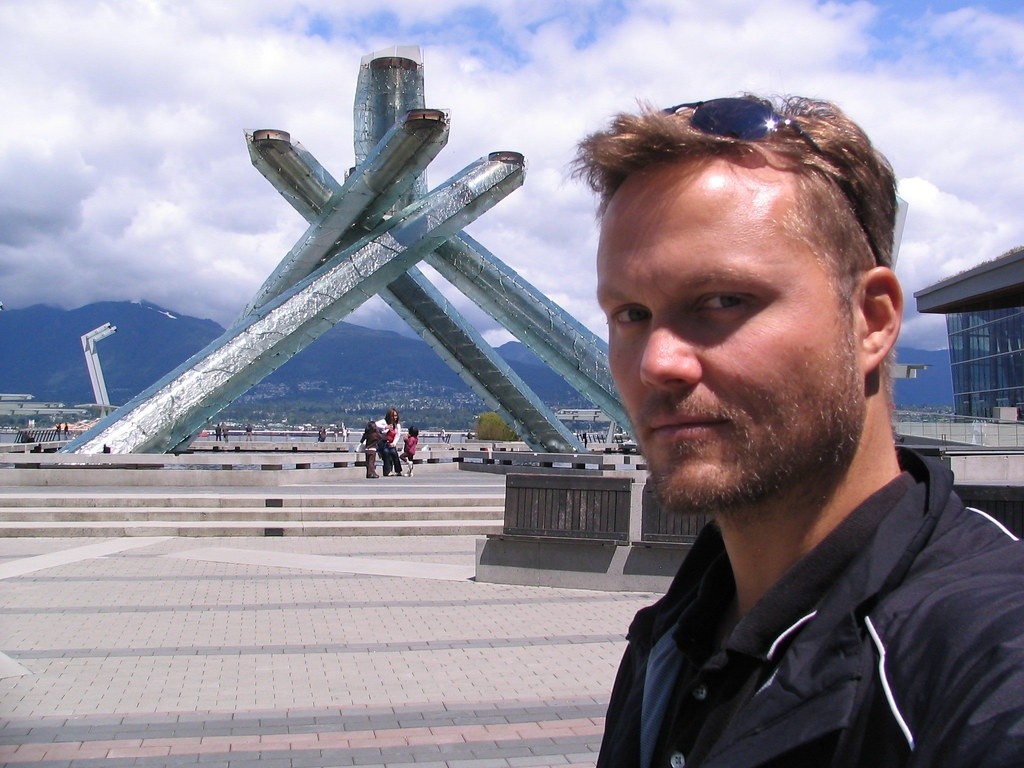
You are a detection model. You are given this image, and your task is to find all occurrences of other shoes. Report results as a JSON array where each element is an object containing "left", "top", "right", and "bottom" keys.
[
  {"left": 388, "top": 472, "right": 394, "bottom": 476},
  {"left": 408, "top": 461, "right": 413, "bottom": 471},
  {"left": 370, "top": 474, "right": 379, "bottom": 478},
  {"left": 404, "top": 473, "right": 413, "bottom": 477},
  {"left": 397, "top": 472, "right": 402, "bottom": 476}
]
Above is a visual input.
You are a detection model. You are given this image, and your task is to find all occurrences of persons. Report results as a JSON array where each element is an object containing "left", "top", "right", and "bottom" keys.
[
  {"left": 56, "top": 423, "right": 62, "bottom": 441},
  {"left": 343, "top": 426, "right": 347, "bottom": 441},
  {"left": 319, "top": 427, "right": 327, "bottom": 442},
  {"left": 441, "top": 427, "right": 445, "bottom": 443},
  {"left": 64, "top": 423, "right": 68, "bottom": 439},
  {"left": 360, "top": 408, "right": 403, "bottom": 478},
  {"left": 215, "top": 424, "right": 229, "bottom": 440},
  {"left": 332, "top": 425, "right": 339, "bottom": 441},
  {"left": 400, "top": 426, "right": 419, "bottom": 476},
  {"left": 246, "top": 424, "right": 252, "bottom": 441},
  {"left": 577, "top": 94, "right": 1024, "bottom": 768}
]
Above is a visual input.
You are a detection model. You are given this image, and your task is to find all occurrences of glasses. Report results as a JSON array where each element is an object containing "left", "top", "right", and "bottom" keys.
[{"left": 660, "top": 97, "right": 887, "bottom": 265}]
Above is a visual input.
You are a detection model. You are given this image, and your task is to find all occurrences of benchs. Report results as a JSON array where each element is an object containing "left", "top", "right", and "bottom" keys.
[
  {"left": 486, "top": 475, "right": 630, "bottom": 546},
  {"left": 632, "top": 480, "right": 713, "bottom": 549}
]
